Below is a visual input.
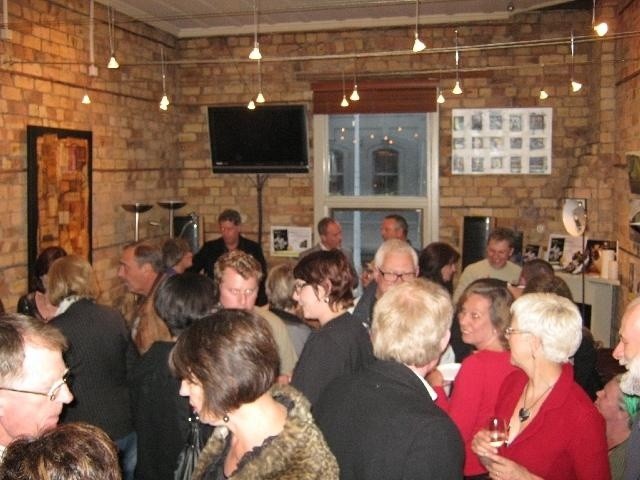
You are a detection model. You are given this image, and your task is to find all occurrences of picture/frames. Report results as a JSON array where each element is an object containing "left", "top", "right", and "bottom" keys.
[{"left": 26, "top": 125, "right": 93, "bottom": 293}]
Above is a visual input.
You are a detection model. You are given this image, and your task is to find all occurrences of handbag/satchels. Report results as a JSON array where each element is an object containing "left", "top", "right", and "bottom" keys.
[{"left": 173, "top": 422, "right": 203, "bottom": 480}]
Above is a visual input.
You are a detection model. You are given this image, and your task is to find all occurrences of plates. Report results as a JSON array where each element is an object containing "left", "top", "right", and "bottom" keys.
[
  {"left": 562, "top": 200, "right": 586, "bottom": 238},
  {"left": 436, "top": 363, "right": 461, "bottom": 380}
]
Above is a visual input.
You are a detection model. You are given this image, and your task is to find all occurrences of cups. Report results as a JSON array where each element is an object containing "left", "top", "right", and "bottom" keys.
[{"left": 601, "top": 250, "right": 619, "bottom": 281}]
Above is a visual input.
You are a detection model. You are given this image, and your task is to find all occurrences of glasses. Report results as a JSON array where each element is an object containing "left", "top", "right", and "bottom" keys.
[
  {"left": 0, "top": 378, "right": 67, "bottom": 401},
  {"left": 505, "top": 328, "right": 534, "bottom": 339},
  {"left": 296, "top": 282, "right": 309, "bottom": 292},
  {"left": 379, "top": 268, "right": 416, "bottom": 281}
]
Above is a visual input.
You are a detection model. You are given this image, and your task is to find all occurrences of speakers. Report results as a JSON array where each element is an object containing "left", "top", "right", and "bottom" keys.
[
  {"left": 462, "top": 216, "right": 490, "bottom": 272},
  {"left": 174, "top": 216, "right": 200, "bottom": 254}
]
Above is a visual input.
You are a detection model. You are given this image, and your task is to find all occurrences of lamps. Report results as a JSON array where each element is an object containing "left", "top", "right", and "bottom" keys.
[
  {"left": 159, "top": 200, "right": 187, "bottom": 239},
  {"left": 563, "top": 200, "right": 588, "bottom": 326},
  {"left": 123, "top": 204, "right": 153, "bottom": 241}
]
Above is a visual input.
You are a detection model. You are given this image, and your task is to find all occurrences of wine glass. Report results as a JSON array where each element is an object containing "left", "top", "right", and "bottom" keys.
[{"left": 487, "top": 417, "right": 506, "bottom": 456}]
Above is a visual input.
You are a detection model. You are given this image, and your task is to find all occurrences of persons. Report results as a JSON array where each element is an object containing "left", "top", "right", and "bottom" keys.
[
  {"left": 574, "top": 202, "right": 586, "bottom": 233},
  {"left": 2, "top": 209, "right": 638, "bottom": 480}
]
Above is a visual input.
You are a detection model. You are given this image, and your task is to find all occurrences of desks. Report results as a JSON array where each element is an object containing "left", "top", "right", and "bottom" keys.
[{"left": 554, "top": 272, "right": 618, "bottom": 348}]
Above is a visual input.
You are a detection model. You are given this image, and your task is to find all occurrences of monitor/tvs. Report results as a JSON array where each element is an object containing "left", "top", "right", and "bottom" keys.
[{"left": 206, "top": 105, "right": 309, "bottom": 174}]
